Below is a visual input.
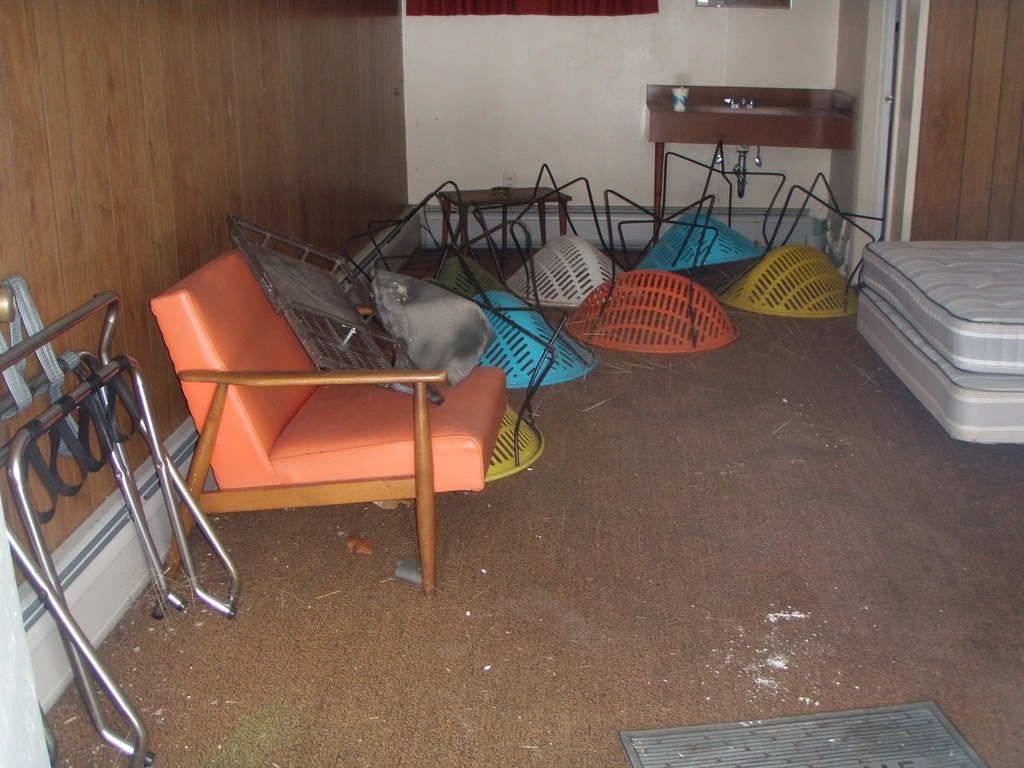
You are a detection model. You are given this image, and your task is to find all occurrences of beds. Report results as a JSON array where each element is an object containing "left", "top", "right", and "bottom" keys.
[{"left": 858, "top": 241, "right": 1024, "bottom": 444}]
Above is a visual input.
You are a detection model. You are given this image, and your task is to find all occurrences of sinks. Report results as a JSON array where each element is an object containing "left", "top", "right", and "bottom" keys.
[{"left": 697, "top": 104, "right": 797, "bottom": 116}]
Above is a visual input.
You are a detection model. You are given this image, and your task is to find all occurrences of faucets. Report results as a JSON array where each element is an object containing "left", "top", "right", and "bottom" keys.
[
  {"left": 740, "top": 96, "right": 756, "bottom": 109},
  {"left": 723, "top": 98, "right": 740, "bottom": 108}
]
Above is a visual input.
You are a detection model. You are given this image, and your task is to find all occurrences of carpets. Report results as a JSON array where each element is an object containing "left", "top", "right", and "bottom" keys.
[{"left": 619, "top": 701, "right": 989, "bottom": 768}]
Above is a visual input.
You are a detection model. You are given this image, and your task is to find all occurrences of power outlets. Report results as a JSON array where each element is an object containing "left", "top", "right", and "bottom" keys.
[{"left": 503, "top": 171, "right": 516, "bottom": 188}]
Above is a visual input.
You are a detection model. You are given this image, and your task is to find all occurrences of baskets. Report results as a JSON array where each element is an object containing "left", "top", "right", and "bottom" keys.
[
  {"left": 633, "top": 213, "right": 765, "bottom": 271},
  {"left": 710, "top": 247, "right": 861, "bottom": 319},
  {"left": 472, "top": 291, "right": 597, "bottom": 388},
  {"left": 565, "top": 270, "right": 741, "bottom": 354},
  {"left": 504, "top": 235, "right": 628, "bottom": 308},
  {"left": 483, "top": 404, "right": 543, "bottom": 484},
  {"left": 438, "top": 257, "right": 508, "bottom": 300}
]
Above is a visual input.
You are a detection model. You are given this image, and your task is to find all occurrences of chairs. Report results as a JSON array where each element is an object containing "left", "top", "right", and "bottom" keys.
[{"left": 150, "top": 249, "right": 506, "bottom": 595}]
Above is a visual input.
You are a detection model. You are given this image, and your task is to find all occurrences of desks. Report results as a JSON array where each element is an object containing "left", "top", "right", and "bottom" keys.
[{"left": 439, "top": 187, "right": 573, "bottom": 257}]
